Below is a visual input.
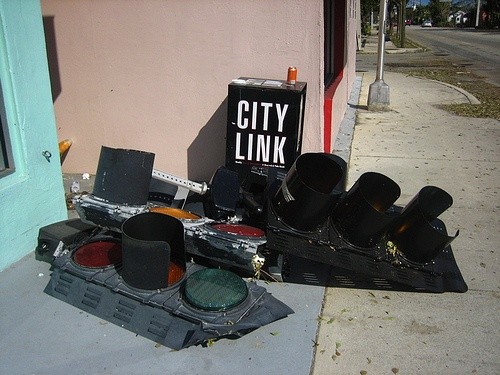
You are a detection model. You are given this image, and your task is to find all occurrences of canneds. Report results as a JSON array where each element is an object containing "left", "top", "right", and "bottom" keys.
[{"left": 287, "top": 66, "right": 297, "bottom": 85}]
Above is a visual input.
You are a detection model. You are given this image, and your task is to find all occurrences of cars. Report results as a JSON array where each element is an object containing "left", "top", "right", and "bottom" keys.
[
  {"left": 404, "top": 18, "right": 411, "bottom": 26},
  {"left": 421, "top": 20, "right": 432, "bottom": 27}
]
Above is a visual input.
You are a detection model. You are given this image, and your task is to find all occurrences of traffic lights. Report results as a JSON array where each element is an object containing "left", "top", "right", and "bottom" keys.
[
  {"left": 266, "top": 151, "right": 460, "bottom": 267},
  {"left": 69, "top": 208, "right": 250, "bottom": 313},
  {"left": 67, "top": 146, "right": 265, "bottom": 272}
]
